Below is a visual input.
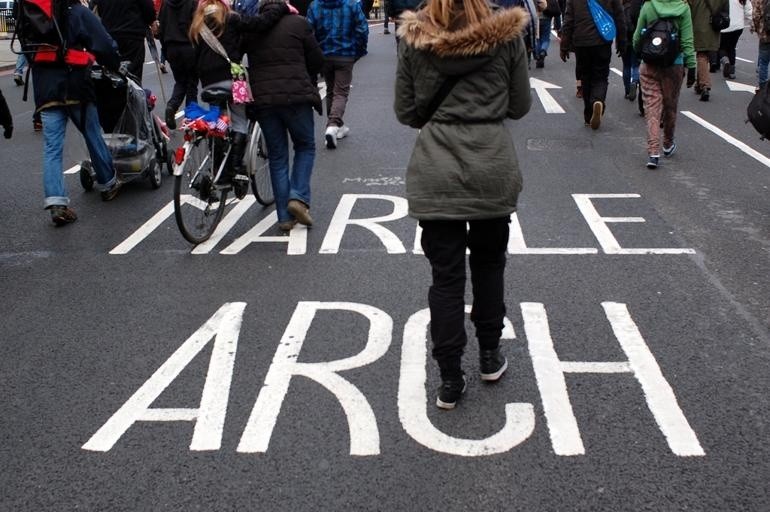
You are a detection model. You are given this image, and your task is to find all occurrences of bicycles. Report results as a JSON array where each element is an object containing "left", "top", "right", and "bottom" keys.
[{"left": 174, "top": 77, "right": 282, "bottom": 244}]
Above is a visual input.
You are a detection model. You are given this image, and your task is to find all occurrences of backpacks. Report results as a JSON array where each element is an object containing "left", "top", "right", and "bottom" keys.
[
  {"left": 12, "top": 0, "right": 96, "bottom": 104},
  {"left": 639, "top": 18, "right": 683, "bottom": 67}
]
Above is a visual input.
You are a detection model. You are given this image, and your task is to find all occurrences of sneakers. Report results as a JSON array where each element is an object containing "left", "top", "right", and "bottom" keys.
[
  {"left": 13, "top": 73, "right": 25, "bottom": 87},
  {"left": 480, "top": 344, "right": 508, "bottom": 381},
  {"left": 437, "top": 375, "right": 467, "bottom": 410},
  {"left": 535, "top": 52, "right": 735, "bottom": 168}
]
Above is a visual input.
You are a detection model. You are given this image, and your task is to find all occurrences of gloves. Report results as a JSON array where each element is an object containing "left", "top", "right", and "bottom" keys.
[
  {"left": 616, "top": 41, "right": 627, "bottom": 56},
  {"left": 560, "top": 49, "right": 569, "bottom": 62}
]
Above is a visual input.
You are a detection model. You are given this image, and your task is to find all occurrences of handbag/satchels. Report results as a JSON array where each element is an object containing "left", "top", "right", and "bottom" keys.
[
  {"left": 589, "top": 1, "right": 616, "bottom": 41},
  {"left": 233, "top": 65, "right": 254, "bottom": 104},
  {"left": 747, "top": 80, "right": 770, "bottom": 139},
  {"left": 712, "top": 10, "right": 730, "bottom": 32}
]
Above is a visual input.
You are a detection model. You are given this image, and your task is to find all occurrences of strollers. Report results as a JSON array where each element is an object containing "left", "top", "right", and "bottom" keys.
[{"left": 79, "top": 60, "right": 177, "bottom": 193}]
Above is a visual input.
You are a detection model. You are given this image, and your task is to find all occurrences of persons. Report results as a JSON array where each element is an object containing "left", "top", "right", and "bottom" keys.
[
  {"left": 0, "top": 0, "right": 770, "bottom": 170},
  {"left": 12, "top": 0, "right": 123, "bottom": 225},
  {"left": 392, "top": 2, "right": 533, "bottom": 411},
  {"left": 187, "top": 1, "right": 255, "bottom": 186},
  {"left": 243, "top": 0, "right": 325, "bottom": 235}
]
